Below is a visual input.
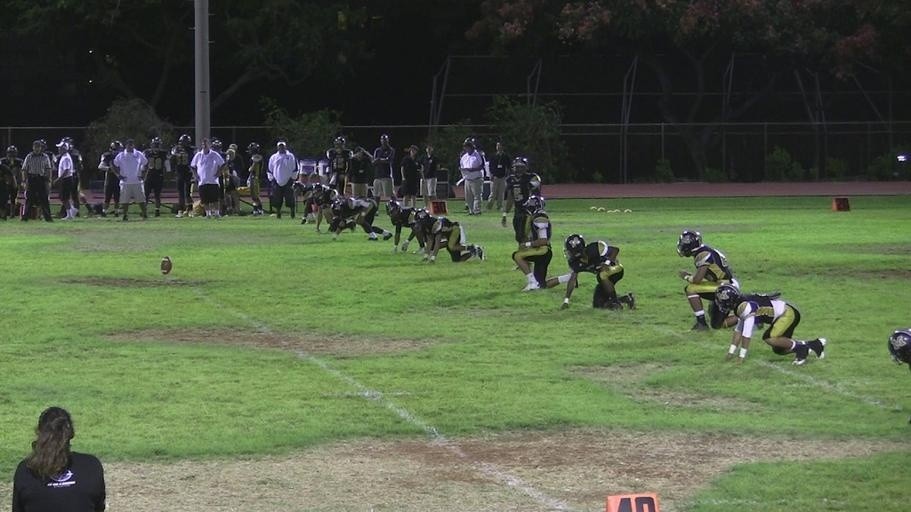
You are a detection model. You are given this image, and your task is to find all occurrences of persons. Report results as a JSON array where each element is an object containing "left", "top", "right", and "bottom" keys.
[
  {"left": 385, "top": 200, "right": 483, "bottom": 262},
  {"left": 1, "top": 136, "right": 96, "bottom": 220},
  {"left": 459, "top": 136, "right": 487, "bottom": 214},
  {"left": 99, "top": 134, "right": 263, "bottom": 222},
  {"left": 486, "top": 141, "right": 508, "bottom": 209},
  {"left": 292, "top": 181, "right": 393, "bottom": 240},
  {"left": 501, "top": 157, "right": 541, "bottom": 271},
  {"left": 512, "top": 195, "right": 578, "bottom": 291},
  {"left": 325, "top": 135, "right": 440, "bottom": 211},
  {"left": 677, "top": 230, "right": 738, "bottom": 330},
  {"left": 561, "top": 233, "right": 635, "bottom": 312},
  {"left": 267, "top": 141, "right": 300, "bottom": 218},
  {"left": 12, "top": 408, "right": 105, "bottom": 512},
  {"left": 715, "top": 284, "right": 825, "bottom": 366}
]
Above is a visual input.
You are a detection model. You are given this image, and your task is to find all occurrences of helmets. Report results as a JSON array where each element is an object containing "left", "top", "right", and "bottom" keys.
[
  {"left": 676, "top": 229, "right": 703, "bottom": 258},
  {"left": 5, "top": 135, "right": 75, "bottom": 154},
  {"left": 109, "top": 134, "right": 287, "bottom": 151},
  {"left": 291, "top": 181, "right": 344, "bottom": 212},
  {"left": 463, "top": 138, "right": 475, "bottom": 149},
  {"left": 714, "top": 282, "right": 742, "bottom": 316},
  {"left": 334, "top": 137, "right": 345, "bottom": 145},
  {"left": 522, "top": 194, "right": 546, "bottom": 216},
  {"left": 385, "top": 199, "right": 430, "bottom": 225},
  {"left": 563, "top": 233, "right": 586, "bottom": 264},
  {"left": 510, "top": 156, "right": 529, "bottom": 175}
]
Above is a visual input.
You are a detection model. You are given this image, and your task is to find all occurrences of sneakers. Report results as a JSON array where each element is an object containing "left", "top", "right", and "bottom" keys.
[
  {"left": 623, "top": 292, "right": 635, "bottom": 310},
  {"left": 464, "top": 203, "right": 504, "bottom": 216},
  {"left": 368, "top": 237, "right": 378, "bottom": 240},
  {"left": 469, "top": 243, "right": 476, "bottom": 257},
  {"left": 1, "top": 207, "right": 294, "bottom": 219},
  {"left": 477, "top": 247, "right": 484, "bottom": 260},
  {"left": 690, "top": 320, "right": 709, "bottom": 331},
  {"left": 815, "top": 338, "right": 827, "bottom": 360},
  {"left": 383, "top": 233, "right": 392, "bottom": 240},
  {"left": 521, "top": 281, "right": 541, "bottom": 293},
  {"left": 791, "top": 340, "right": 812, "bottom": 366}
]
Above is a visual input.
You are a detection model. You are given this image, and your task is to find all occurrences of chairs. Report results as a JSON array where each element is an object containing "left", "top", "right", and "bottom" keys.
[{"left": 435, "top": 169, "right": 452, "bottom": 199}]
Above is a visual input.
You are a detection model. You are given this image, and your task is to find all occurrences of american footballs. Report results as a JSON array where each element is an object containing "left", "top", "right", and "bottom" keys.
[{"left": 161, "top": 256, "right": 170, "bottom": 275}]
